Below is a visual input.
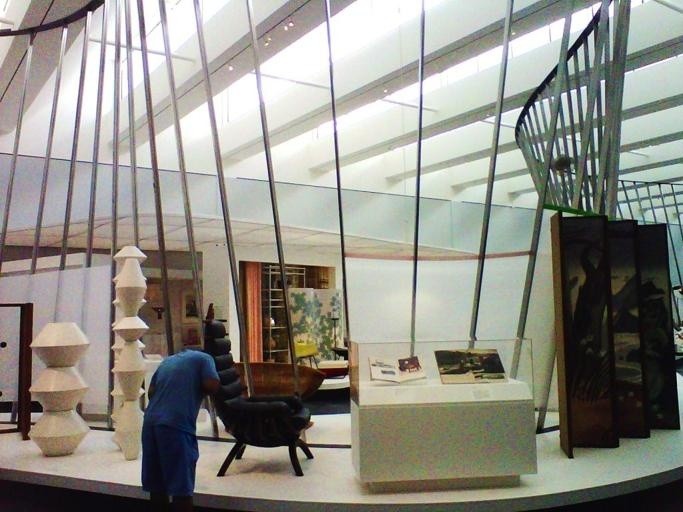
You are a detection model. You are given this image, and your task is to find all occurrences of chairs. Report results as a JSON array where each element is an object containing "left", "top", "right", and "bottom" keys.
[
  {"left": 293, "top": 337, "right": 319, "bottom": 369},
  {"left": 200, "top": 316, "right": 315, "bottom": 479}
]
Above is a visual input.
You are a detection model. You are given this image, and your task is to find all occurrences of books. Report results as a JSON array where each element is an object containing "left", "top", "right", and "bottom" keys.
[
  {"left": 368, "top": 354, "right": 425, "bottom": 383},
  {"left": 434, "top": 348, "right": 506, "bottom": 385}
]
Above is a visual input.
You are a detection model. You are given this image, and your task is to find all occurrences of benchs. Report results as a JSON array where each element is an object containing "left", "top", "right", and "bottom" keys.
[{"left": 317, "top": 360, "right": 348, "bottom": 378}]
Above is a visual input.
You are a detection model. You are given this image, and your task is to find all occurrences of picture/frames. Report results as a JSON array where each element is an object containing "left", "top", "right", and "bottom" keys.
[{"left": 179, "top": 288, "right": 202, "bottom": 348}]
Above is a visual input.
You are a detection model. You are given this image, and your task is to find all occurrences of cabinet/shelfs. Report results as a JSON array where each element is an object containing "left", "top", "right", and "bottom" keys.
[{"left": 246, "top": 262, "right": 306, "bottom": 362}]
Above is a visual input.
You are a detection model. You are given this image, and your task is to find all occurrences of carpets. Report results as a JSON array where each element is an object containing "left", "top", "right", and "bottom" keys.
[{"left": 298, "top": 387, "right": 350, "bottom": 414}]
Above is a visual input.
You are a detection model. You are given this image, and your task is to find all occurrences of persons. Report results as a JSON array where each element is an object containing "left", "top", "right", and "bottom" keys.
[{"left": 139, "top": 323, "right": 220, "bottom": 512}]
